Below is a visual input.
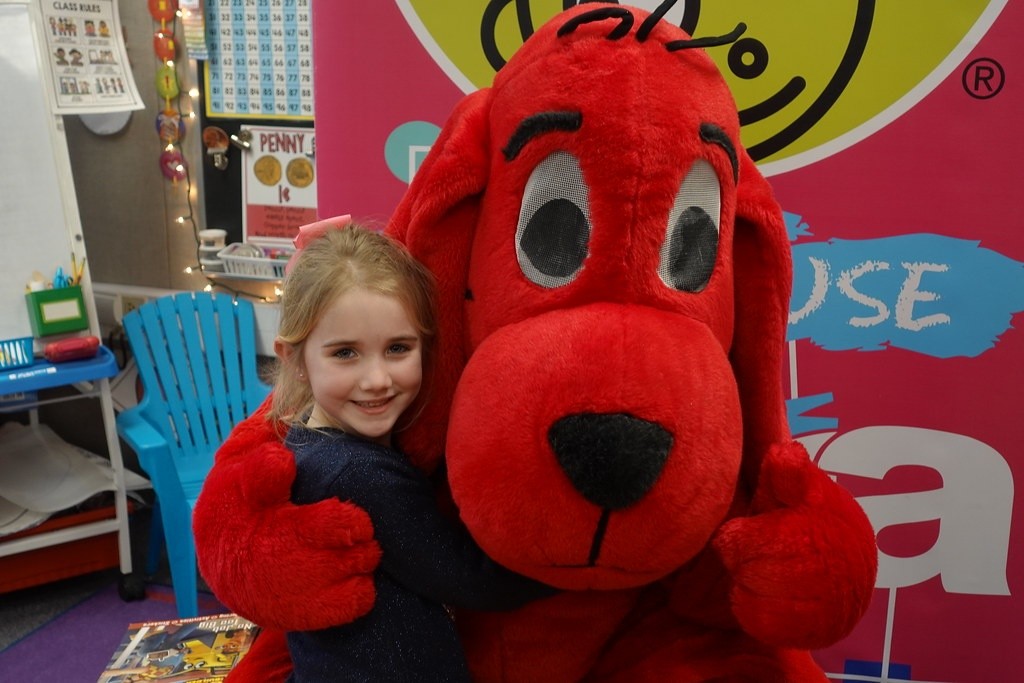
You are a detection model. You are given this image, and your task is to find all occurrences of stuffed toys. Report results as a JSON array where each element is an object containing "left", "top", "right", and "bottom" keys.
[{"left": 191, "top": 8, "right": 877, "bottom": 683}]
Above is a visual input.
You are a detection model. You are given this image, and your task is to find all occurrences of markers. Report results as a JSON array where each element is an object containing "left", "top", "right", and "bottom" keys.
[{"left": 53, "top": 267, "right": 62, "bottom": 289}]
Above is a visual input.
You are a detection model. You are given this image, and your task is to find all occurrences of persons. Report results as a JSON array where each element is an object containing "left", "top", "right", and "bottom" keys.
[{"left": 277, "top": 223, "right": 569, "bottom": 683}]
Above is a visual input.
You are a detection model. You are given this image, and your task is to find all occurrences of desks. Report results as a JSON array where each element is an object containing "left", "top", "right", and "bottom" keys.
[{"left": 0, "top": 346, "right": 146, "bottom": 603}]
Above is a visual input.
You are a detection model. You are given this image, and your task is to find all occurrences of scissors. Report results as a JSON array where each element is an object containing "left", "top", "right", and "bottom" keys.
[{"left": 54, "top": 275, "right": 72, "bottom": 287}]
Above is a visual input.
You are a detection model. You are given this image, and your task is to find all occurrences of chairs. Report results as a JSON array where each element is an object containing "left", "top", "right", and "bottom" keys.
[{"left": 115, "top": 289, "right": 271, "bottom": 618}]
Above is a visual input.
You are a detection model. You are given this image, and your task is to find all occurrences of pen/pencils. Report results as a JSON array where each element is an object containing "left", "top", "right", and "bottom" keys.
[{"left": 70, "top": 251, "right": 85, "bottom": 286}]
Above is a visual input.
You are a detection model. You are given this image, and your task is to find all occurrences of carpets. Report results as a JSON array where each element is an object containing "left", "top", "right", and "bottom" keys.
[{"left": 0, "top": 578, "right": 265, "bottom": 683}]
